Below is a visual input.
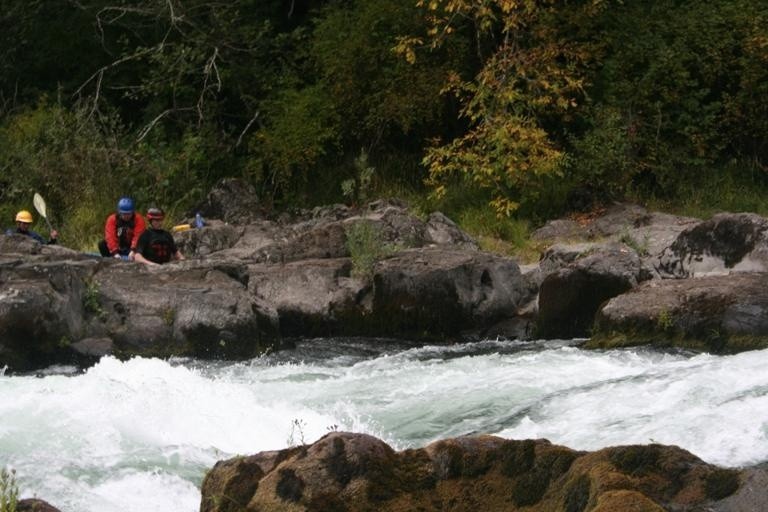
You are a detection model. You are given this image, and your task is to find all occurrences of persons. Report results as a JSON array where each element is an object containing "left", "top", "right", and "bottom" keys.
[
  {"left": 135, "top": 204, "right": 186, "bottom": 264},
  {"left": 5, "top": 210, "right": 58, "bottom": 244},
  {"left": 97, "top": 199, "right": 145, "bottom": 262}
]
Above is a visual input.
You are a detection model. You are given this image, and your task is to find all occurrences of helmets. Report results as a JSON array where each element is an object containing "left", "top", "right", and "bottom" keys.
[
  {"left": 147, "top": 208, "right": 165, "bottom": 220},
  {"left": 117, "top": 198, "right": 134, "bottom": 214},
  {"left": 16, "top": 210, "right": 33, "bottom": 223}
]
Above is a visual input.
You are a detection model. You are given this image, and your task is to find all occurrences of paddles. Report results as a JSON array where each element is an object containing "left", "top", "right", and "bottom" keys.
[{"left": 32, "top": 194, "right": 53, "bottom": 232}]
